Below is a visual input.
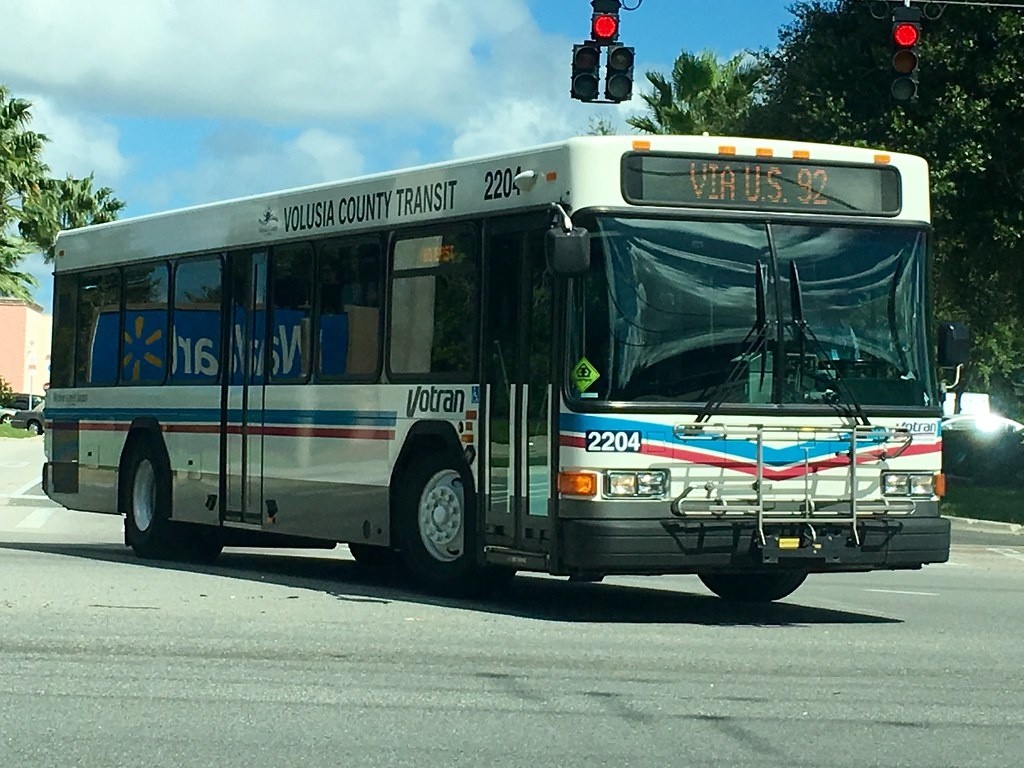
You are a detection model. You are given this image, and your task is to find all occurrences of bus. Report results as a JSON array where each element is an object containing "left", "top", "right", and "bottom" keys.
[{"left": 42, "top": 135, "right": 970, "bottom": 600}]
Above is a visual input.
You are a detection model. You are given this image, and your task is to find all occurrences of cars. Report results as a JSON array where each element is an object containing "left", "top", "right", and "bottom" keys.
[
  {"left": 941, "top": 413, "right": 1024, "bottom": 473},
  {"left": 0, "top": 405, "right": 20, "bottom": 424},
  {"left": 0, "top": 394, "right": 46, "bottom": 412},
  {"left": 11, "top": 411, "right": 45, "bottom": 435}
]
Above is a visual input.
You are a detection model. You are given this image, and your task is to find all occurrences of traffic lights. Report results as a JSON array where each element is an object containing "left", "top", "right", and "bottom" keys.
[
  {"left": 888, "top": 6, "right": 919, "bottom": 104},
  {"left": 571, "top": 41, "right": 601, "bottom": 101},
  {"left": 590, "top": 13, "right": 619, "bottom": 41},
  {"left": 604, "top": 43, "right": 636, "bottom": 101}
]
